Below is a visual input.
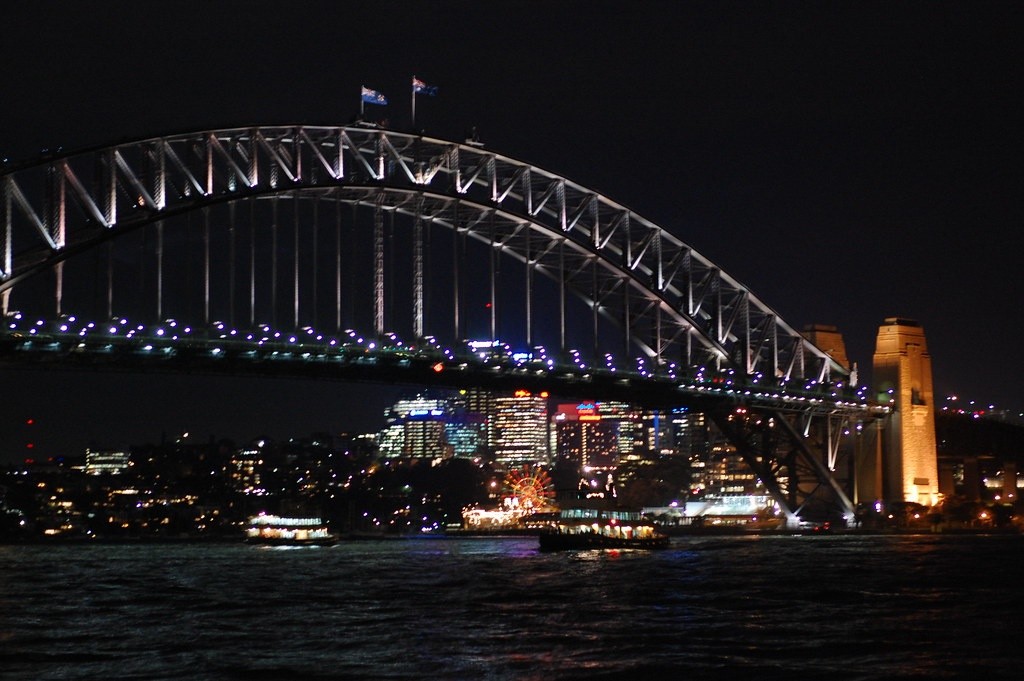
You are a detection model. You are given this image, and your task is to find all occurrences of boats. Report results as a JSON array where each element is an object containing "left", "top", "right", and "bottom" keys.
[
  {"left": 243, "top": 512, "right": 339, "bottom": 547},
  {"left": 533, "top": 520, "right": 671, "bottom": 554}
]
